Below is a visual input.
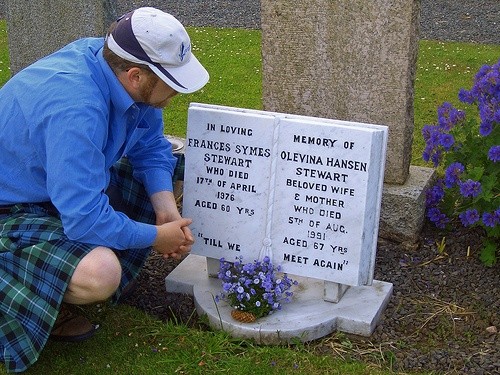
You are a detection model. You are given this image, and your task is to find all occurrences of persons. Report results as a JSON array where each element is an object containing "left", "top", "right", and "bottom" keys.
[{"left": 0, "top": 7, "right": 210, "bottom": 375}]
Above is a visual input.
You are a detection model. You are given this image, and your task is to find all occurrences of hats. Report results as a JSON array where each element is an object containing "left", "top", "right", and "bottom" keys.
[{"left": 105, "top": 6, "right": 209, "bottom": 94}]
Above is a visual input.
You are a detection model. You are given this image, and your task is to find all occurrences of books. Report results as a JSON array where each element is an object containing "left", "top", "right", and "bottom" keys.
[{"left": 181, "top": 102, "right": 388, "bottom": 288}]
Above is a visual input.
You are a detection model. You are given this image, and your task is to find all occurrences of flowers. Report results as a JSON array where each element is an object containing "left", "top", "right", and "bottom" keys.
[{"left": 213, "top": 254, "right": 300, "bottom": 319}]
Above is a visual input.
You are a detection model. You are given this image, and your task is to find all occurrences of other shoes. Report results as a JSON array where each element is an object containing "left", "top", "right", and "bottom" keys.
[{"left": 51, "top": 307, "right": 96, "bottom": 342}]
